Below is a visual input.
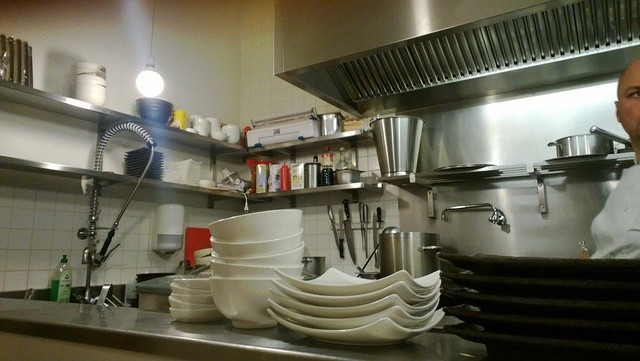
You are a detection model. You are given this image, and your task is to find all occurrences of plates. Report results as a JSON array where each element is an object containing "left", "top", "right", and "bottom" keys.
[
  {"left": 168, "top": 158, "right": 203, "bottom": 187},
  {"left": 266, "top": 265, "right": 447, "bottom": 348},
  {"left": 168, "top": 274, "right": 224, "bottom": 324},
  {"left": 499, "top": 163, "right": 528, "bottom": 176},
  {"left": 435, "top": 248, "right": 638, "bottom": 359},
  {"left": 122, "top": 146, "right": 165, "bottom": 183},
  {"left": 432, "top": 162, "right": 497, "bottom": 173}
]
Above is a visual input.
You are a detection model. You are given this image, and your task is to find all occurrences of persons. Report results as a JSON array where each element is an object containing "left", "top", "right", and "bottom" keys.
[{"left": 590, "top": 60, "right": 640, "bottom": 259}]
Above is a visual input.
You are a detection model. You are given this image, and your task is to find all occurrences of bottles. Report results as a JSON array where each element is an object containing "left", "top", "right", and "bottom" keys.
[
  {"left": 279, "top": 160, "right": 290, "bottom": 192},
  {"left": 320, "top": 146, "right": 335, "bottom": 186}
]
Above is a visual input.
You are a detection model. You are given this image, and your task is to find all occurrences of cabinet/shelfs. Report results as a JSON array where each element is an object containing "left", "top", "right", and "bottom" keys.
[
  {"left": 0, "top": 80, "right": 266, "bottom": 202},
  {"left": 216, "top": 129, "right": 383, "bottom": 199}
]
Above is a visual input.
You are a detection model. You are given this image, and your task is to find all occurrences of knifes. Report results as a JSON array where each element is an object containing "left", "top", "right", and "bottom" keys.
[
  {"left": 337, "top": 208, "right": 344, "bottom": 260},
  {"left": 371, "top": 212, "right": 381, "bottom": 269},
  {"left": 342, "top": 198, "right": 357, "bottom": 266}
]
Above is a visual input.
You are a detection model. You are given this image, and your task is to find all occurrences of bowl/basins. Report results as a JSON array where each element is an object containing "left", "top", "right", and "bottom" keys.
[
  {"left": 334, "top": 167, "right": 366, "bottom": 186},
  {"left": 174, "top": 109, "right": 192, "bottom": 133},
  {"left": 207, "top": 207, "right": 306, "bottom": 332},
  {"left": 135, "top": 96, "right": 173, "bottom": 126},
  {"left": 319, "top": 111, "right": 346, "bottom": 139},
  {"left": 192, "top": 115, "right": 240, "bottom": 148},
  {"left": 71, "top": 60, "right": 110, "bottom": 111}
]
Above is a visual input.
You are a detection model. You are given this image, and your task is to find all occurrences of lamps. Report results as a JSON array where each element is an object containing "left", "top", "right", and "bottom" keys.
[{"left": 136, "top": 0, "right": 165, "bottom": 98}]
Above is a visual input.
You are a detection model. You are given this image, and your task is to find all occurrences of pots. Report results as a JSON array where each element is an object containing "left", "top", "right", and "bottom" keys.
[
  {"left": 378, "top": 226, "right": 441, "bottom": 281},
  {"left": 545, "top": 134, "right": 616, "bottom": 156}
]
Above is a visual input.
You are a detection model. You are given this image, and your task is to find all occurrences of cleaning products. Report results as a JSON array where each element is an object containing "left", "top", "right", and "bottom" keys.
[{"left": 50, "top": 253, "right": 71, "bottom": 303}]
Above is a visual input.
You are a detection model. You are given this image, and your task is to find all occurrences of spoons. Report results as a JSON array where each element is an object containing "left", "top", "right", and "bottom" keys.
[{"left": 326, "top": 205, "right": 339, "bottom": 254}]
